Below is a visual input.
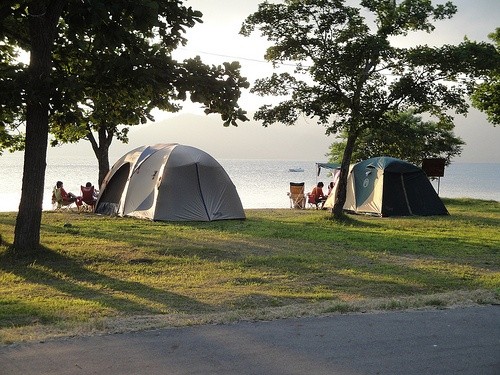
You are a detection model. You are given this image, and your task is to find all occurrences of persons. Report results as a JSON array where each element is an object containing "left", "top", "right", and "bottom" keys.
[
  {"left": 56, "top": 181, "right": 79, "bottom": 211},
  {"left": 86, "top": 182, "right": 98, "bottom": 202},
  {"left": 312, "top": 182, "right": 326, "bottom": 210},
  {"left": 327, "top": 181, "right": 335, "bottom": 196}
]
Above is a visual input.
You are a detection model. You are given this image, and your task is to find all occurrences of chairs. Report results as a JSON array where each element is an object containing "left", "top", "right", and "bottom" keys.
[
  {"left": 308, "top": 194, "right": 323, "bottom": 210},
  {"left": 287, "top": 182, "right": 308, "bottom": 209},
  {"left": 77, "top": 186, "right": 95, "bottom": 213},
  {"left": 53, "top": 188, "right": 73, "bottom": 213}
]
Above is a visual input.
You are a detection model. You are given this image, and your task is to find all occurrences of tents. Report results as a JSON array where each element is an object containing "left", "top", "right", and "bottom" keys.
[
  {"left": 95, "top": 142, "right": 247, "bottom": 222},
  {"left": 322, "top": 156, "right": 452, "bottom": 217}
]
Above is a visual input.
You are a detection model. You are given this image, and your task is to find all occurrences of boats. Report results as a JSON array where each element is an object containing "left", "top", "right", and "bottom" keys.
[{"left": 289, "top": 168, "right": 304, "bottom": 172}]
